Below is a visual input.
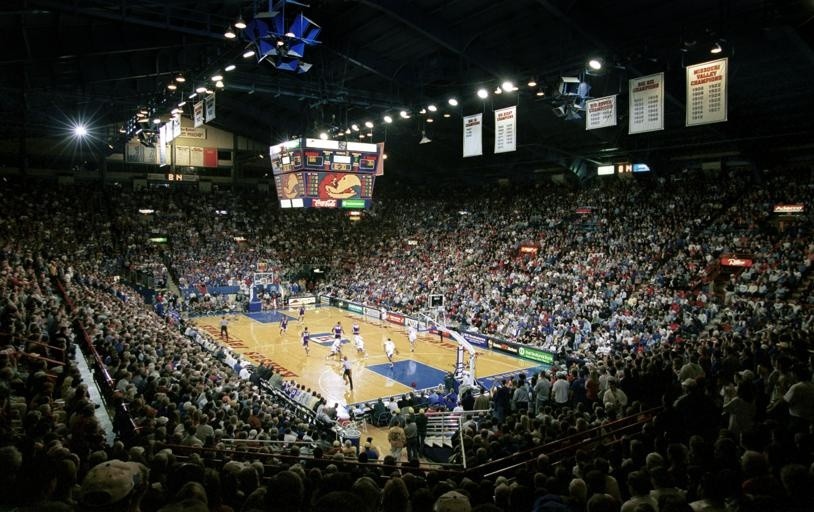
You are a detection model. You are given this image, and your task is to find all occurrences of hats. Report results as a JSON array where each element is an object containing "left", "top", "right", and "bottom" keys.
[
  {"left": 432, "top": 490, "right": 472, "bottom": 511},
  {"left": 80, "top": 458, "right": 149, "bottom": 503}
]
